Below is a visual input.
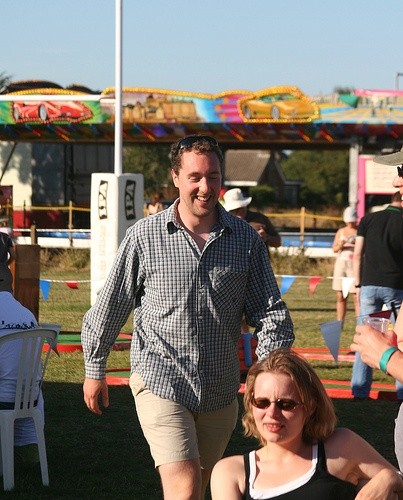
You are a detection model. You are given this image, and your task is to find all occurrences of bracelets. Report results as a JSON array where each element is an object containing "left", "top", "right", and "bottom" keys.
[{"left": 380, "top": 346, "right": 400, "bottom": 373}]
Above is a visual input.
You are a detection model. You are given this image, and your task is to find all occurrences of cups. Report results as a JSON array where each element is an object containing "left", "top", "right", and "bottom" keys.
[{"left": 362, "top": 317, "right": 393, "bottom": 344}]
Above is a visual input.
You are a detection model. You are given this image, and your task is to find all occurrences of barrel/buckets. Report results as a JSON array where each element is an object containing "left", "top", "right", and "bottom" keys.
[{"left": 237, "top": 333, "right": 258, "bottom": 370}]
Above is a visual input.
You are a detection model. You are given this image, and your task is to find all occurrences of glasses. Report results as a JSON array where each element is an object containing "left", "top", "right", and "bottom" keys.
[
  {"left": 250, "top": 396, "right": 306, "bottom": 411},
  {"left": 397, "top": 166, "right": 403, "bottom": 179},
  {"left": 176, "top": 135, "right": 218, "bottom": 155}
]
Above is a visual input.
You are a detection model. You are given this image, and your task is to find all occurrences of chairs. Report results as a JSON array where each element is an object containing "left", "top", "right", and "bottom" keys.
[{"left": 0, "top": 327, "right": 59, "bottom": 490}]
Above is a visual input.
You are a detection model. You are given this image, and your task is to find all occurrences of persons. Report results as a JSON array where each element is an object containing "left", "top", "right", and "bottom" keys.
[
  {"left": 332, "top": 206, "right": 359, "bottom": 320},
  {"left": 222, "top": 188, "right": 281, "bottom": 335},
  {"left": 0, "top": 261, "right": 44, "bottom": 447},
  {"left": 145, "top": 192, "right": 163, "bottom": 216},
  {"left": 80, "top": 135, "right": 295, "bottom": 500},
  {"left": 349, "top": 165, "right": 403, "bottom": 474},
  {"left": 0, "top": 231, "right": 15, "bottom": 267},
  {"left": 349, "top": 191, "right": 403, "bottom": 401},
  {"left": 211, "top": 346, "right": 402, "bottom": 500}
]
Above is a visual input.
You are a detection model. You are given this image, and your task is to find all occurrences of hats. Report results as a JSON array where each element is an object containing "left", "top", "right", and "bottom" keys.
[
  {"left": 223, "top": 187, "right": 253, "bottom": 212},
  {"left": 373, "top": 145, "right": 403, "bottom": 166},
  {"left": 343, "top": 206, "right": 357, "bottom": 223}
]
